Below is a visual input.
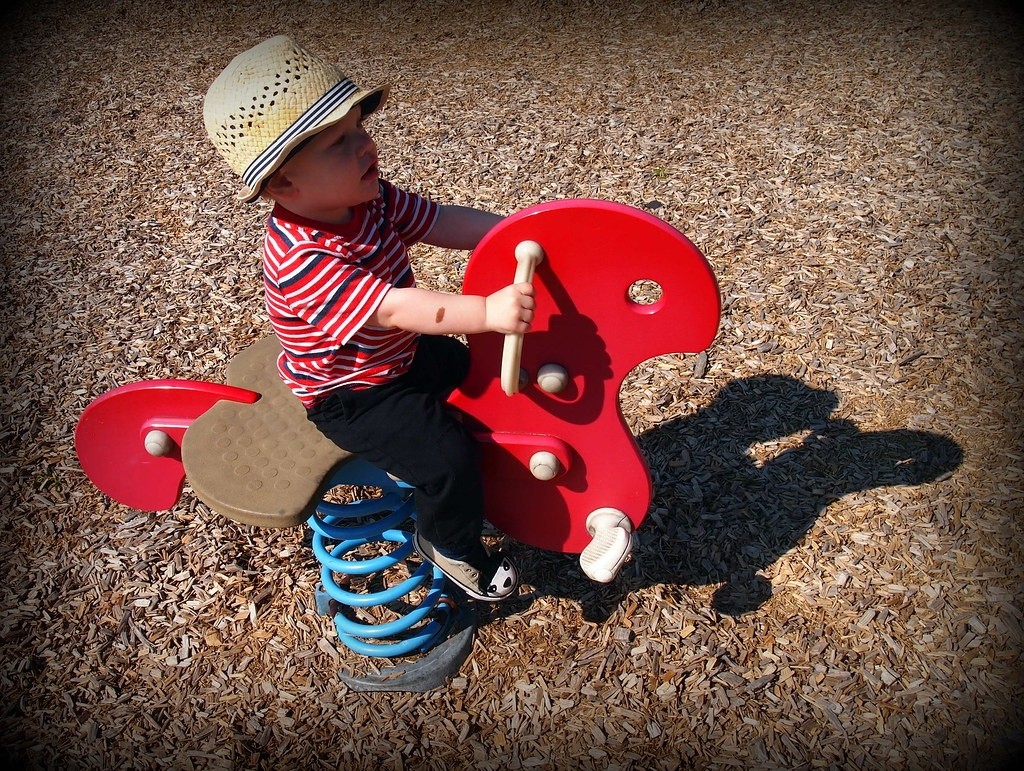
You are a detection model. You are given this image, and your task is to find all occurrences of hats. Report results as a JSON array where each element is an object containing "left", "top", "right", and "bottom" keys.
[{"left": 202, "top": 31, "right": 391, "bottom": 206}]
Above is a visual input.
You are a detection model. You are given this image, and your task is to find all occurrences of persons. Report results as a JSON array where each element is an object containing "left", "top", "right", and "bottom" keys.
[{"left": 201, "top": 35, "right": 533, "bottom": 603}]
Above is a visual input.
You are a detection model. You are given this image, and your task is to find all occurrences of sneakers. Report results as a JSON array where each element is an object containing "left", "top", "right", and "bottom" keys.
[{"left": 409, "top": 532, "right": 521, "bottom": 603}]
